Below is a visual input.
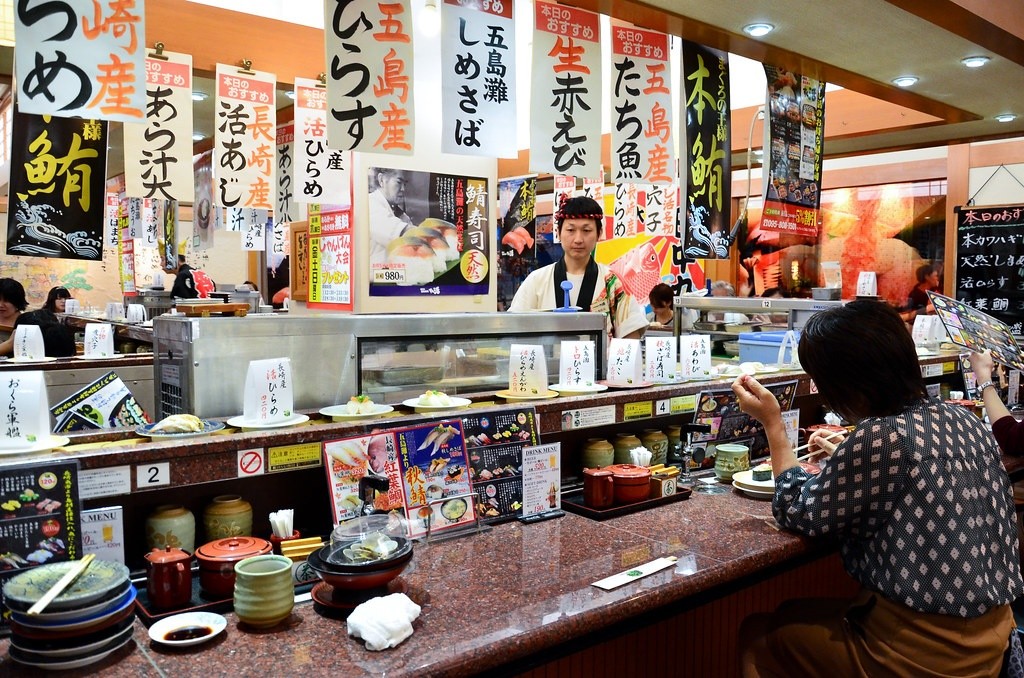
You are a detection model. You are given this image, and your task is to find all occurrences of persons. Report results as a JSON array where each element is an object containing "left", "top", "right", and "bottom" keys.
[
  {"left": 732, "top": 296, "right": 1024, "bottom": 678},
  {"left": 0, "top": 278, "right": 35, "bottom": 359},
  {"left": 368, "top": 167, "right": 416, "bottom": 284},
  {"left": 695, "top": 281, "right": 750, "bottom": 327},
  {"left": 158, "top": 238, "right": 216, "bottom": 313},
  {"left": 497, "top": 273, "right": 505, "bottom": 312},
  {"left": 905, "top": 265, "right": 940, "bottom": 325},
  {"left": 33, "top": 286, "right": 77, "bottom": 357},
  {"left": 506, "top": 195, "right": 649, "bottom": 360},
  {"left": 645, "top": 282, "right": 684, "bottom": 327},
  {"left": 243, "top": 281, "right": 265, "bottom": 305},
  {"left": 751, "top": 289, "right": 789, "bottom": 325}
]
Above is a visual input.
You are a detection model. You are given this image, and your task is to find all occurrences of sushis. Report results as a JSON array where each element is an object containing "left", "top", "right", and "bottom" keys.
[
  {"left": 1, "top": 488, "right": 66, "bottom": 570},
  {"left": 149, "top": 414, "right": 204, "bottom": 433},
  {"left": 418, "top": 390, "right": 454, "bottom": 406},
  {"left": 712, "top": 362, "right": 765, "bottom": 374},
  {"left": 385, "top": 217, "right": 460, "bottom": 285},
  {"left": 752, "top": 463, "right": 772, "bottom": 482},
  {"left": 327, "top": 441, "right": 369, "bottom": 467},
  {"left": 346, "top": 394, "right": 376, "bottom": 415},
  {"left": 501, "top": 226, "right": 534, "bottom": 254},
  {"left": 416, "top": 423, "right": 530, "bottom": 516},
  {"left": 0, "top": 434, "right": 66, "bottom": 450}
]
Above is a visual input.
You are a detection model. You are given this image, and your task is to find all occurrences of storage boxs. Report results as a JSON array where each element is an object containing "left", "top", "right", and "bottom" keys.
[{"left": 738, "top": 330, "right": 802, "bottom": 364}]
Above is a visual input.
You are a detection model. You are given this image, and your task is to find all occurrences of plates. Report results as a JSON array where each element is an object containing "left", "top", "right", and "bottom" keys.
[
  {"left": 6, "top": 355, "right": 125, "bottom": 362},
  {"left": 0, "top": 436, "right": 70, "bottom": 455},
  {"left": 311, "top": 576, "right": 409, "bottom": 609},
  {"left": 3, "top": 560, "right": 137, "bottom": 670},
  {"left": 176, "top": 299, "right": 224, "bottom": 313},
  {"left": 136, "top": 365, "right": 801, "bottom": 441},
  {"left": 63, "top": 313, "right": 153, "bottom": 327},
  {"left": 732, "top": 460, "right": 821, "bottom": 498},
  {"left": 148, "top": 612, "right": 227, "bottom": 646}
]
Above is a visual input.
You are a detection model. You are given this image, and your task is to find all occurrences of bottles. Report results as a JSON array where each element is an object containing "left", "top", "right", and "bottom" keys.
[
  {"left": 584, "top": 439, "right": 615, "bottom": 469},
  {"left": 614, "top": 434, "right": 642, "bottom": 464},
  {"left": 550, "top": 482, "right": 556, "bottom": 507},
  {"left": 642, "top": 429, "right": 668, "bottom": 466},
  {"left": 148, "top": 495, "right": 253, "bottom": 553},
  {"left": 668, "top": 425, "right": 682, "bottom": 463}
]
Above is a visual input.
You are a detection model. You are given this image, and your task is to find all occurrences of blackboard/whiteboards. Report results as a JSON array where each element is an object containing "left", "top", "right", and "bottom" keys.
[{"left": 952, "top": 202, "right": 1024, "bottom": 339}]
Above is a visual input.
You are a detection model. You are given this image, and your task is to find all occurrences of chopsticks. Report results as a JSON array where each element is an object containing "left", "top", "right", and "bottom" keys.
[
  {"left": 767, "top": 430, "right": 848, "bottom": 461},
  {"left": 27, "top": 553, "right": 96, "bottom": 616}
]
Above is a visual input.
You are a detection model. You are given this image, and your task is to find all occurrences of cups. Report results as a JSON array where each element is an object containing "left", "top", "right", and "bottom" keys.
[
  {"left": 144, "top": 546, "right": 193, "bottom": 608},
  {"left": 584, "top": 464, "right": 614, "bottom": 507},
  {"left": 550, "top": 455, "right": 555, "bottom": 468},
  {"left": 270, "top": 530, "right": 299, "bottom": 555},
  {"left": 102, "top": 525, "right": 113, "bottom": 543},
  {"left": 416, "top": 507, "right": 435, "bottom": 528},
  {"left": 198, "top": 198, "right": 210, "bottom": 229},
  {"left": 233, "top": 554, "right": 295, "bottom": 628},
  {"left": 950, "top": 391, "right": 964, "bottom": 401},
  {"left": 715, "top": 444, "right": 749, "bottom": 481}
]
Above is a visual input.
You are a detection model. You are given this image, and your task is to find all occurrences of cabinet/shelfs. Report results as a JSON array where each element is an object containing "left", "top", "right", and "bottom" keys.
[{"left": 0, "top": 349, "right": 1024, "bottom": 678}]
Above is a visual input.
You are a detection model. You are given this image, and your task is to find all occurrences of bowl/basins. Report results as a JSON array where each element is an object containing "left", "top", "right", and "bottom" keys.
[
  {"left": 604, "top": 464, "right": 651, "bottom": 501},
  {"left": 307, "top": 514, "right": 414, "bottom": 587},
  {"left": 723, "top": 341, "right": 739, "bottom": 356},
  {"left": 441, "top": 497, "right": 468, "bottom": 522},
  {"left": 768, "top": 313, "right": 788, "bottom": 323},
  {"left": 194, "top": 536, "right": 273, "bottom": 596},
  {"left": 702, "top": 400, "right": 717, "bottom": 413}
]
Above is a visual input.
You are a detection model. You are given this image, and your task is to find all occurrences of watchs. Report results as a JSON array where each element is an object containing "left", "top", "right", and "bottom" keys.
[{"left": 977, "top": 381, "right": 994, "bottom": 399}]
